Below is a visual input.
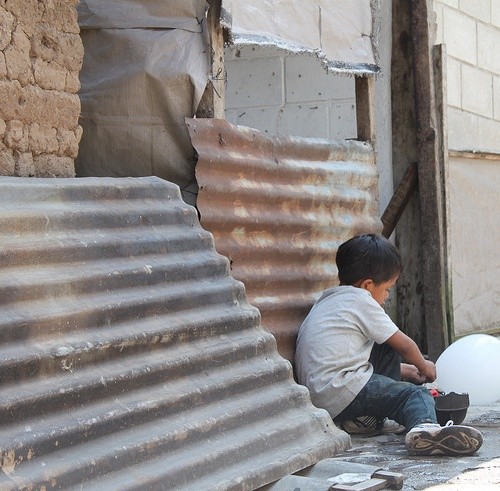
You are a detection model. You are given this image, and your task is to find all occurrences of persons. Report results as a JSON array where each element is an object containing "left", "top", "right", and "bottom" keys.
[{"left": 295, "top": 234, "right": 483, "bottom": 457}]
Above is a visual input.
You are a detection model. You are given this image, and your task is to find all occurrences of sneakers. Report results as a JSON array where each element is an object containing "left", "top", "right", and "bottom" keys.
[
  {"left": 342, "top": 415, "right": 406, "bottom": 434},
  {"left": 405, "top": 422, "right": 483, "bottom": 457}
]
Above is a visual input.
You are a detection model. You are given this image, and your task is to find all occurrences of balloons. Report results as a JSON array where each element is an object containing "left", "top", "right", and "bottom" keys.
[{"left": 433, "top": 334, "right": 500, "bottom": 406}]
